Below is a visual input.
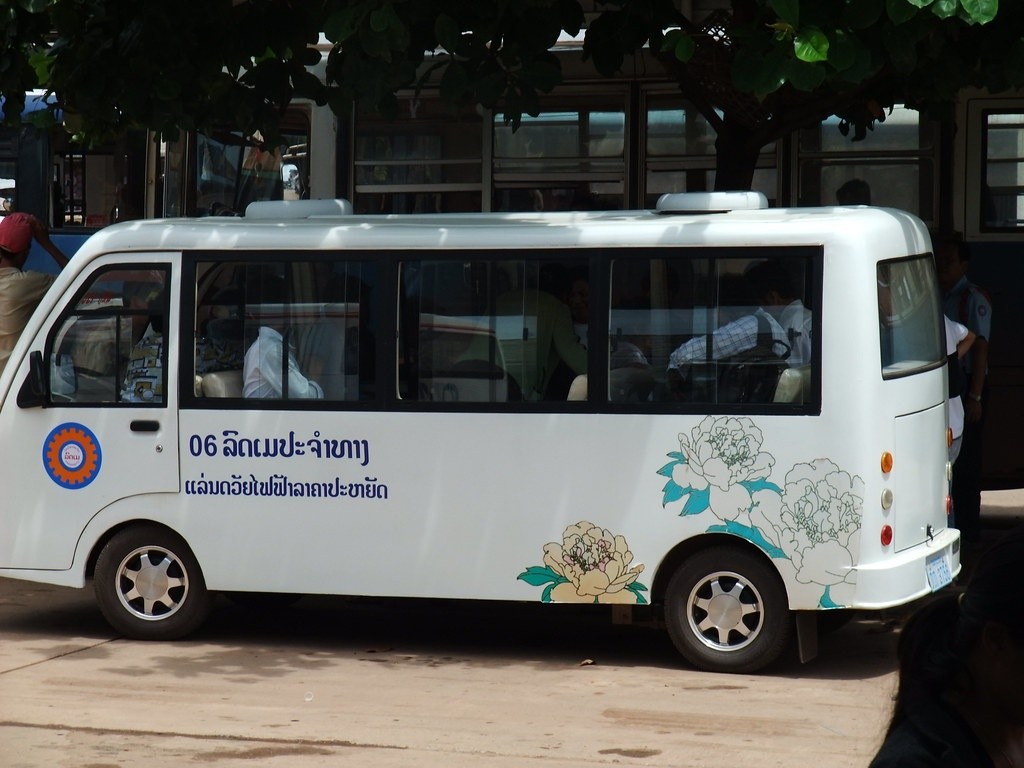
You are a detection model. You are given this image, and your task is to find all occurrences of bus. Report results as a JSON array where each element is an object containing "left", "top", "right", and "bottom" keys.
[{"left": 0, "top": 28, "right": 1024, "bottom": 371}]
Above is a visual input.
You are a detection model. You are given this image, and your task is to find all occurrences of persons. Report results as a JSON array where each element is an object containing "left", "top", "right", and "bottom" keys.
[
  {"left": 73, "top": 177, "right": 1024, "bottom": 768},
  {"left": 0, "top": 213, "right": 69, "bottom": 378}
]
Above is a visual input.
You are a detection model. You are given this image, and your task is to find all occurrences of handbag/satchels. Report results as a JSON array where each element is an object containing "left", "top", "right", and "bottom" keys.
[{"left": 718, "top": 314, "right": 792, "bottom": 404}]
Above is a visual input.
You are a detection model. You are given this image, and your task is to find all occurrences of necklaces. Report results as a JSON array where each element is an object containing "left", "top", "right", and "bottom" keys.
[{"left": 948, "top": 688, "right": 1015, "bottom": 768}]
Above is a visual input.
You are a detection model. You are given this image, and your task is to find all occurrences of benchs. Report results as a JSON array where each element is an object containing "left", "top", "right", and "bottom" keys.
[
  {"left": 195, "top": 369, "right": 244, "bottom": 398},
  {"left": 775, "top": 364, "right": 809, "bottom": 405},
  {"left": 567, "top": 363, "right": 646, "bottom": 401}
]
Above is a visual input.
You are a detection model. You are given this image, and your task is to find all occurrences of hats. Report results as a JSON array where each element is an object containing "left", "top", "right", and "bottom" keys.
[{"left": 0, "top": 212, "right": 33, "bottom": 253}]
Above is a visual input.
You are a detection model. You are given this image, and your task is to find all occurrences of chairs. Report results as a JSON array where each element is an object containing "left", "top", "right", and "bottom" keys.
[{"left": 450, "top": 359, "right": 523, "bottom": 403}]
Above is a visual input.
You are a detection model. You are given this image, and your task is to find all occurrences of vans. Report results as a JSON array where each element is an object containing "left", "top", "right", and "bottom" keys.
[{"left": 2, "top": 190, "right": 962, "bottom": 674}]
